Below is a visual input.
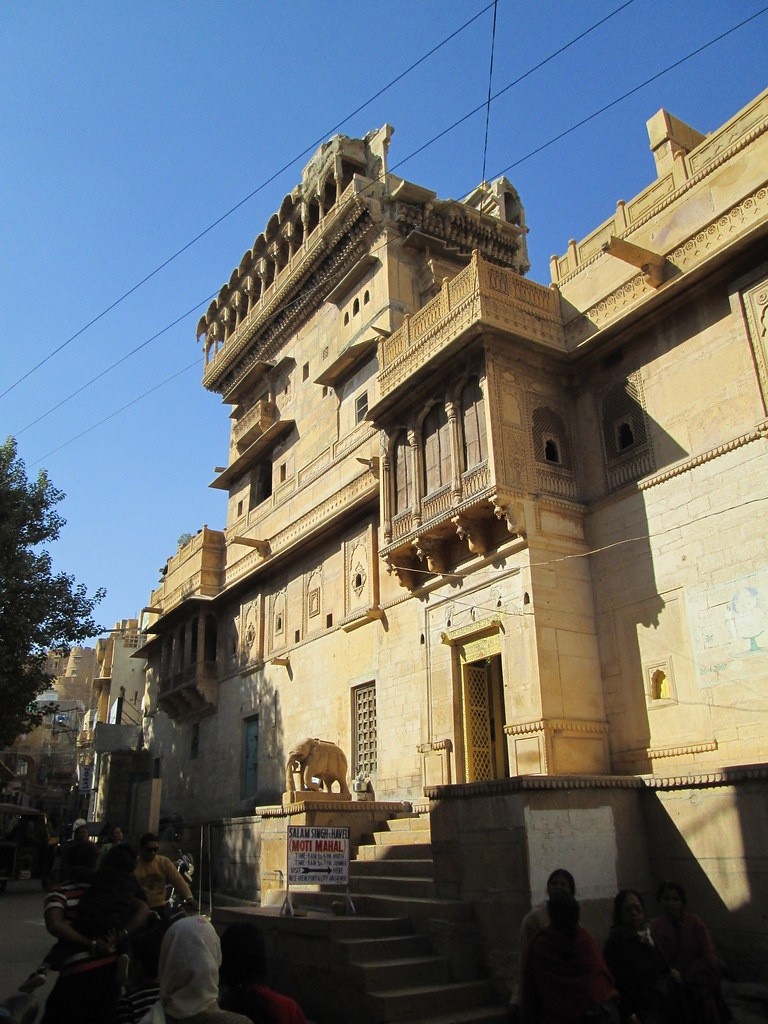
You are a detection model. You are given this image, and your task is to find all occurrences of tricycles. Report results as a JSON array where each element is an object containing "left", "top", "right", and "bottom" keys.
[{"left": 0, "top": 804, "right": 60, "bottom": 892}]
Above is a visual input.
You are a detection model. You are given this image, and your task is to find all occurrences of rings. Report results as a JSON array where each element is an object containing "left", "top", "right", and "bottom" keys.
[{"left": 187, "top": 896, "right": 192, "bottom": 900}]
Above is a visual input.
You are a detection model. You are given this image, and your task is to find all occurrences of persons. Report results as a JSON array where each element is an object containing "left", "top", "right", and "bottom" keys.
[
  {"left": 652, "top": 884, "right": 732, "bottom": 1024},
  {"left": 604, "top": 890, "right": 666, "bottom": 1024},
  {"left": 18, "top": 824, "right": 201, "bottom": 1024},
  {"left": 508, "top": 870, "right": 621, "bottom": 1024},
  {"left": 220, "top": 922, "right": 307, "bottom": 1024},
  {"left": 140, "top": 915, "right": 254, "bottom": 1024}
]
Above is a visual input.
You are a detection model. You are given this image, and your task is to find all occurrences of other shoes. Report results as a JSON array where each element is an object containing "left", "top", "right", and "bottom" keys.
[
  {"left": 18, "top": 969, "right": 46, "bottom": 993},
  {"left": 115, "top": 954, "right": 128, "bottom": 986}
]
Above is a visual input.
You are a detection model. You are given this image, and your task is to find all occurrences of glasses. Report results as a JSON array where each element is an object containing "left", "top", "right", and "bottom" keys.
[{"left": 145, "top": 847, "right": 158, "bottom": 853}]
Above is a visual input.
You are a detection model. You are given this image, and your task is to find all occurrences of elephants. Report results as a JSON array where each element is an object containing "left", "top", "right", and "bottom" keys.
[{"left": 284, "top": 738, "right": 350, "bottom": 793}]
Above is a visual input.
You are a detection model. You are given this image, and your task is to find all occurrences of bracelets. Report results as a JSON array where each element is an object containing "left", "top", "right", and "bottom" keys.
[{"left": 630, "top": 1014, "right": 637, "bottom": 1020}]
